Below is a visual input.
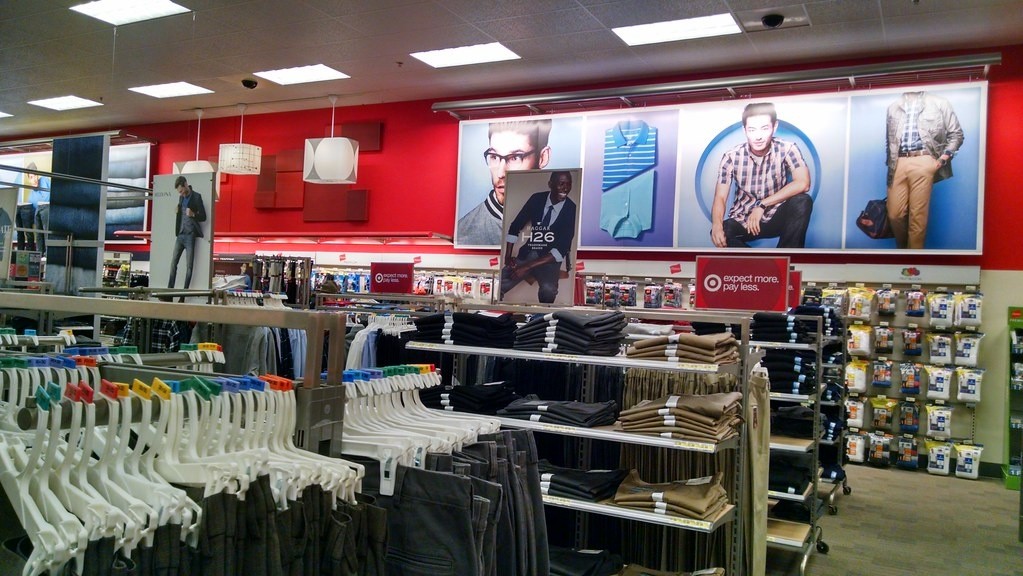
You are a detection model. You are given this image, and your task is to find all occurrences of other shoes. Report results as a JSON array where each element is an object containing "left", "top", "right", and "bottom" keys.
[
  {"left": 178, "top": 297, "right": 186, "bottom": 303},
  {"left": 157, "top": 295, "right": 175, "bottom": 302}
]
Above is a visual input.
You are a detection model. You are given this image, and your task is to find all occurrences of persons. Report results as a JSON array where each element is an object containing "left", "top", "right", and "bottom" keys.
[
  {"left": 885, "top": 90, "right": 964, "bottom": 249},
  {"left": 457, "top": 119, "right": 552, "bottom": 245},
  {"left": 710, "top": 102, "right": 813, "bottom": 248},
  {"left": 27, "top": 162, "right": 50, "bottom": 226},
  {"left": 330, "top": 275, "right": 336, "bottom": 285},
  {"left": 15, "top": 203, "right": 35, "bottom": 251},
  {"left": 158, "top": 176, "right": 206, "bottom": 302},
  {"left": 500, "top": 171, "right": 576, "bottom": 303},
  {"left": 35, "top": 202, "right": 50, "bottom": 255}
]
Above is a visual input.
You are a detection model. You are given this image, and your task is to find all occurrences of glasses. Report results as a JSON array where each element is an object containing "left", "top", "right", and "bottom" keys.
[{"left": 485, "top": 144, "right": 546, "bottom": 170}]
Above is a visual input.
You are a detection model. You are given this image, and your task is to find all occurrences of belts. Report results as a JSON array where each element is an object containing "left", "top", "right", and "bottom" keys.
[{"left": 897, "top": 150, "right": 931, "bottom": 157}]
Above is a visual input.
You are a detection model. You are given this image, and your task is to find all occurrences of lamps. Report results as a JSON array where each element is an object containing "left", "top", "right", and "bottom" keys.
[
  {"left": 299, "top": 93, "right": 361, "bottom": 187},
  {"left": 171, "top": 107, "right": 221, "bottom": 203},
  {"left": 217, "top": 102, "right": 262, "bottom": 176}
]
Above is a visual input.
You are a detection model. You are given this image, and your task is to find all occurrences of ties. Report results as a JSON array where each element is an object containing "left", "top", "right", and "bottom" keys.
[{"left": 541, "top": 205, "right": 554, "bottom": 230}]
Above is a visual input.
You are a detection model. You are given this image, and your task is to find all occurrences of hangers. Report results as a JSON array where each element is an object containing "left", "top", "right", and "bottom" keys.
[{"left": 0, "top": 293, "right": 505, "bottom": 576}]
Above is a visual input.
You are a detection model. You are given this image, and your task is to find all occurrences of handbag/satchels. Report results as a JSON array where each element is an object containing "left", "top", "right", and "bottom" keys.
[{"left": 857, "top": 199, "right": 897, "bottom": 239}]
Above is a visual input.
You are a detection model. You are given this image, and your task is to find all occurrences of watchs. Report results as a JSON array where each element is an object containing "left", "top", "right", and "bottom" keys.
[
  {"left": 755, "top": 200, "right": 766, "bottom": 209},
  {"left": 937, "top": 158, "right": 946, "bottom": 166}
]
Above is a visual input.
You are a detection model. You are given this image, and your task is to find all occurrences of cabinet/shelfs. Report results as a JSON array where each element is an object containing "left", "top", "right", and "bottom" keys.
[{"left": 392, "top": 283, "right": 854, "bottom": 576}]
